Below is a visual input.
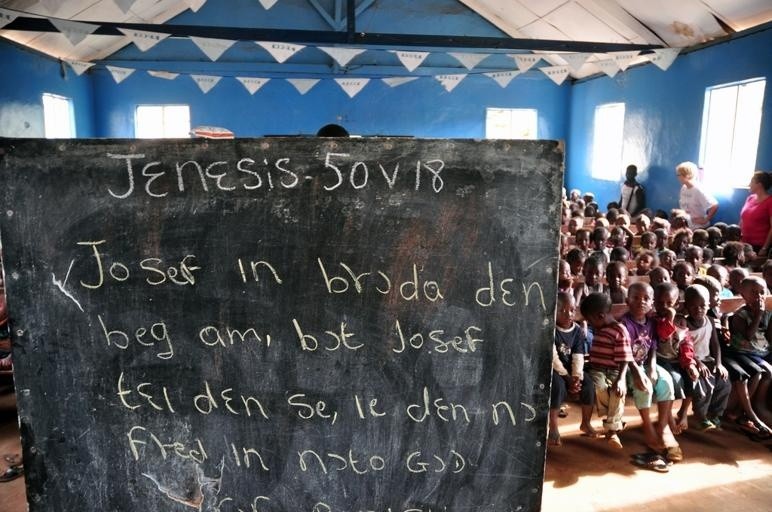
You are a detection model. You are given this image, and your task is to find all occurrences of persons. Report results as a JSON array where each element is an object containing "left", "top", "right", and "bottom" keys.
[
  {"left": 673, "top": 161, "right": 719, "bottom": 231},
  {"left": 737, "top": 172, "right": 771, "bottom": 260},
  {"left": 616, "top": 165, "right": 645, "bottom": 216},
  {"left": 544, "top": 185, "right": 772, "bottom": 468}
]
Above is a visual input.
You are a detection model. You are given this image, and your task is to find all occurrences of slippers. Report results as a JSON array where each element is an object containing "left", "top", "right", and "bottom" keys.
[
  {"left": 559, "top": 404, "right": 570, "bottom": 417},
  {"left": 0, "top": 453, "right": 24, "bottom": 482},
  {"left": 617, "top": 422, "right": 628, "bottom": 434},
  {"left": 608, "top": 431, "right": 623, "bottom": 448},
  {"left": 700, "top": 416, "right": 772, "bottom": 439},
  {"left": 548, "top": 431, "right": 562, "bottom": 448},
  {"left": 632, "top": 445, "right": 684, "bottom": 472},
  {"left": 580, "top": 430, "right": 605, "bottom": 438}
]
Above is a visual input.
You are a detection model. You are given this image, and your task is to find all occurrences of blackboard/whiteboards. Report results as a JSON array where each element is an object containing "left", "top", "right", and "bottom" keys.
[{"left": 0, "top": 137, "right": 565, "bottom": 512}]
